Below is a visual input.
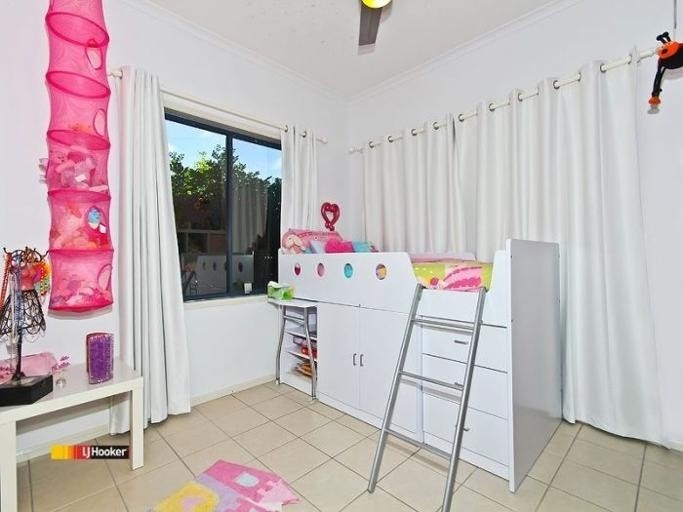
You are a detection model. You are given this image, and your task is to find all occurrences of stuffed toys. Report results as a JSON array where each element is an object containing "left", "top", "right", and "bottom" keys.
[
  {"left": 649, "top": 32, "right": 683, "bottom": 105},
  {"left": 51, "top": 255, "right": 112, "bottom": 307},
  {"left": 81, "top": 204, "right": 110, "bottom": 248},
  {"left": 53, "top": 206, "right": 98, "bottom": 249},
  {"left": 282, "top": 234, "right": 312, "bottom": 256},
  {"left": 49, "top": 149, "right": 98, "bottom": 190}
]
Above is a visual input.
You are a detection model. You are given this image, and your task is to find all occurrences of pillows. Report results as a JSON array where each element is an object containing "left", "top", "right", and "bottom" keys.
[
  {"left": 326, "top": 238, "right": 352, "bottom": 253},
  {"left": 352, "top": 240, "right": 370, "bottom": 252},
  {"left": 309, "top": 240, "right": 324, "bottom": 254},
  {"left": 288, "top": 228, "right": 343, "bottom": 254}
]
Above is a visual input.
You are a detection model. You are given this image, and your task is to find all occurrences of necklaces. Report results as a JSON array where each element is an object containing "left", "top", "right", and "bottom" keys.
[
  {"left": 9, "top": 272, "right": 19, "bottom": 373},
  {"left": 40, "top": 259, "right": 48, "bottom": 295},
  {"left": 0, "top": 252, "right": 11, "bottom": 309}
]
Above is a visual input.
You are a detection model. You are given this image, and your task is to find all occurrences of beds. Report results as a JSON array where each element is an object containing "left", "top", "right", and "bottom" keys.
[{"left": 267, "top": 238, "right": 561, "bottom": 493}]
